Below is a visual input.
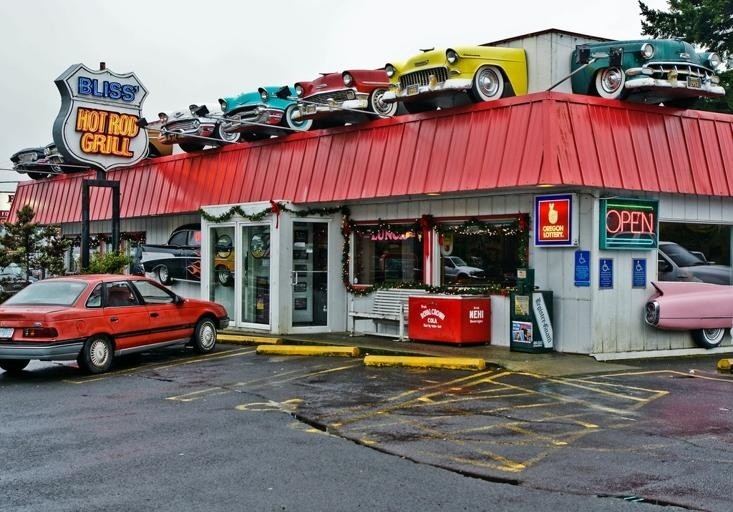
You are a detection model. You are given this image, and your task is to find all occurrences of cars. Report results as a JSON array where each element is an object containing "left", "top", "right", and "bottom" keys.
[
  {"left": 139, "top": 224, "right": 235, "bottom": 287},
  {"left": 571, "top": 39, "right": 726, "bottom": 106},
  {"left": 441, "top": 256, "right": 485, "bottom": 284},
  {"left": 9, "top": 142, "right": 91, "bottom": 180},
  {"left": 281, "top": 69, "right": 398, "bottom": 133},
  {"left": 381, "top": 47, "right": 528, "bottom": 115},
  {"left": 659, "top": 240, "right": 730, "bottom": 283},
  {"left": 136, "top": 86, "right": 315, "bottom": 155},
  {"left": 0, "top": 274, "right": 229, "bottom": 374}
]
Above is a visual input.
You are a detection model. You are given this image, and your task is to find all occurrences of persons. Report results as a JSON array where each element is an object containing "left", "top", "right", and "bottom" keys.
[{"left": 512, "top": 324, "right": 531, "bottom": 342}]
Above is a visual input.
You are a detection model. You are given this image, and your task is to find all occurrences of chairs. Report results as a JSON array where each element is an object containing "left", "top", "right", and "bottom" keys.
[{"left": 107, "top": 286, "right": 131, "bottom": 305}]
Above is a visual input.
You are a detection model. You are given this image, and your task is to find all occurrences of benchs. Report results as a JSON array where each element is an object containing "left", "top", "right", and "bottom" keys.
[{"left": 349, "top": 289, "right": 425, "bottom": 342}]
